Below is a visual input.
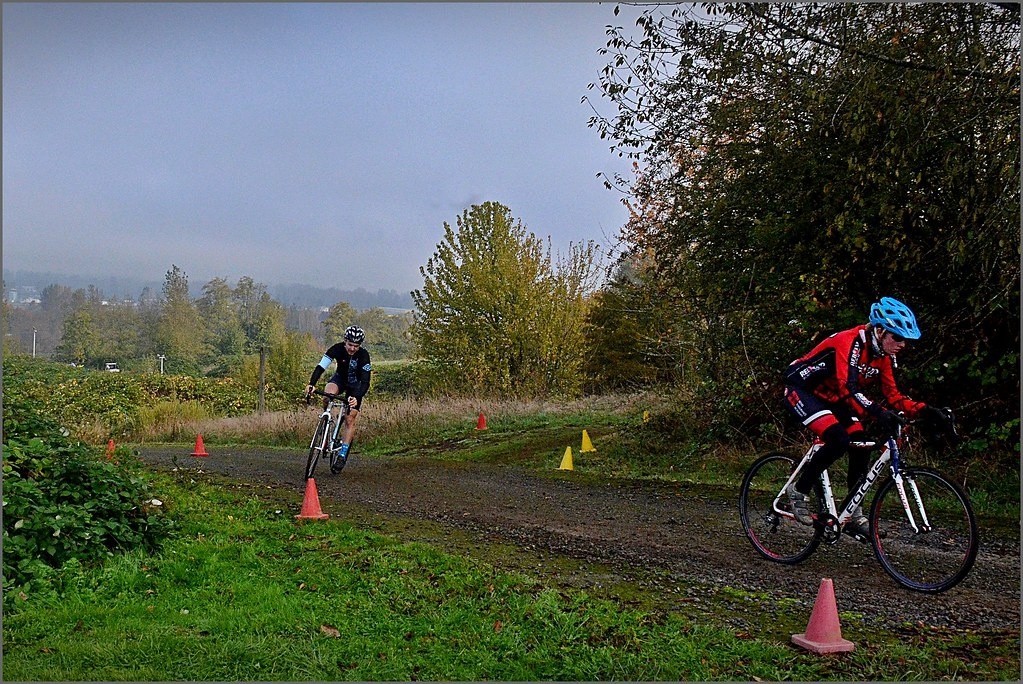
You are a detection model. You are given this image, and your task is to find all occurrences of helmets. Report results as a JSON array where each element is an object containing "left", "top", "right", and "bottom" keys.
[
  {"left": 868, "top": 297, "right": 921, "bottom": 339},
  {"left": 344, "top": 325, "right": 365, "bottom": 343}
]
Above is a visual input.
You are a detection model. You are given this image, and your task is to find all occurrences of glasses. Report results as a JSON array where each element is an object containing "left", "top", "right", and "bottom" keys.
[{"left": 892, "top": 333, "right": 904, "bottom": 342}]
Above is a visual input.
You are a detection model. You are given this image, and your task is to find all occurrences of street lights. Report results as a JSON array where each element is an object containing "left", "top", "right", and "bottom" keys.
[{"left": 33, "top": 326, "right": 38, "bottom": 357}]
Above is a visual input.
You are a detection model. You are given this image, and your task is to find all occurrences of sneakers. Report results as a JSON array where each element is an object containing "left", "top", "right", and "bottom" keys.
[
  {"left": 844, "top": 515, "right": 887, "bottom": 539},
  {"left": 334, "top": 455, "right": 347, "bottom": 469},
  {"left": 318, "top": 428, "right": 331, "bottom": 435},
  {"left": 785, "top": 480, "right": 814, "bottom": 526}
]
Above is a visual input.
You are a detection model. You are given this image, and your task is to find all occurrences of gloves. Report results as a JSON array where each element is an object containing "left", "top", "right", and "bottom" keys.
[
  {"left": 919, "top": 404, "right": 955, "bottom": 425},
  {"left": 872, "top": 399, "right": 904, "bottom": 425}
]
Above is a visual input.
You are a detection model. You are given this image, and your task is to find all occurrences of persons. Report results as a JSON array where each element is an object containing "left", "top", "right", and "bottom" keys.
[
  {"left": 774, "top": 296, "right": 955, "bottom": 539},
  {"left": 305, "top": 326, "right": 374, "bottom": 471}
]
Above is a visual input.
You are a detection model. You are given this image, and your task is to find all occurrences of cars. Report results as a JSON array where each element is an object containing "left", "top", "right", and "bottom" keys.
[{"left": 157, "top": 353, "right": 166, "bottom": 374}]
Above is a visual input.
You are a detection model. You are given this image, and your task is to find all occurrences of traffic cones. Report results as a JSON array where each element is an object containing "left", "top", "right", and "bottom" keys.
[
  {"left": 555, "top": 446, "right": 574, "bottom": 471},
  {"left": 643, "top": 411, "right": 650, "bottom": 423},
  {"left": 190, "top": 434, "right": 209, "bottom": 456},
  {"left": 791, "top": 578, "right": 855, "bottom": 654},
  {"left": 294, "top": 478, "right": 329, "bottom": 521},
  {"left": 473, "top": 412, "right": 491, "bottom": 431},
  {"left": 107, "top": 440, "right": 118, "bottom": 464},
  {"left": 579, "top": 430, "right": 597, "bottom": 453}
]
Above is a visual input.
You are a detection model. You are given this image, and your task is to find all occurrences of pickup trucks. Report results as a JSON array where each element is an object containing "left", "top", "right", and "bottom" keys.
[{"left": 105, "top": 362, "right": 121, "bottom": 372}]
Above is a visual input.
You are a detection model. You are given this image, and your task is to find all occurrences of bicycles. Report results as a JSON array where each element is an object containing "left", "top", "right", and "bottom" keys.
[
  {"left": 738, "top": 407, "right": 979, "bottom": 594},
  {"left": 304, "top": 386, "right": 353, "bottom": 481}
]
